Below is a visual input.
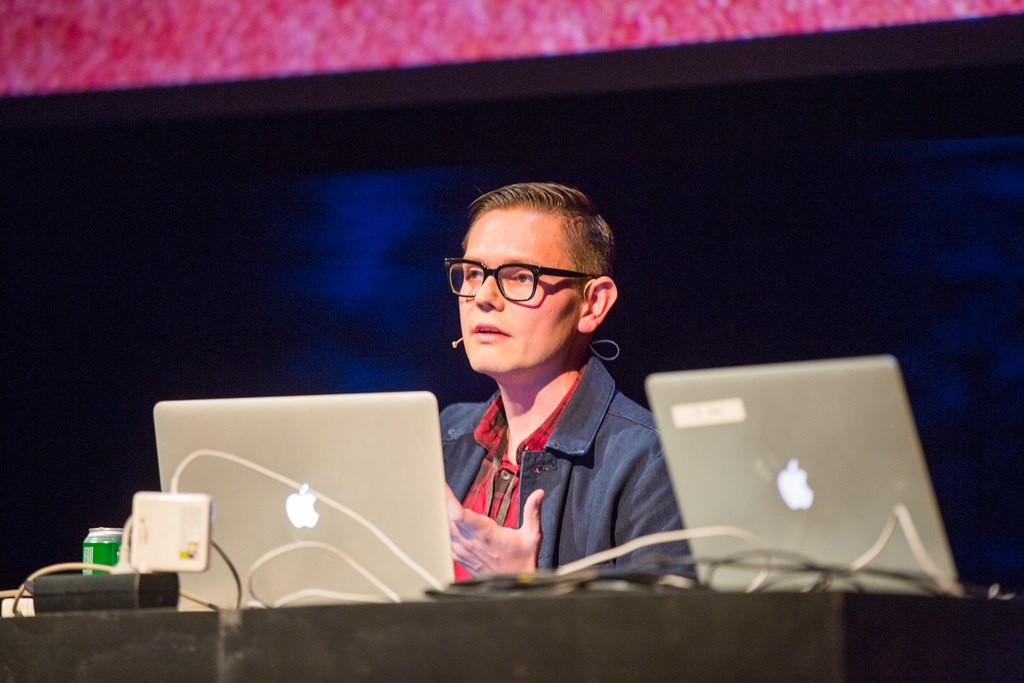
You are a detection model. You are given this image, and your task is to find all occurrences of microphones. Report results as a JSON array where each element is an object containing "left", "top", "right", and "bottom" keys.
[{"left": 452, "top": 337, "right": 463, "bottom": 348}]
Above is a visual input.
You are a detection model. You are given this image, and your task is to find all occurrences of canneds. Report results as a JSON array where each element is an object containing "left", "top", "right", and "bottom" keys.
[{"left": 82, "top": 528, "right": 124, "bottom": 575}]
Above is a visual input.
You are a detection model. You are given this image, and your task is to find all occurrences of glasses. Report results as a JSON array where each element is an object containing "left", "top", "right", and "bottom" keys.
[{"left": 446, "top": 258, "right": 597, "bottom": 302}]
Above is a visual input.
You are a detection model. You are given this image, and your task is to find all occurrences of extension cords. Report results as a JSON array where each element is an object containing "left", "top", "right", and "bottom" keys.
[{"left": 33, "top": 571, "right": 180, "bottom": 615}]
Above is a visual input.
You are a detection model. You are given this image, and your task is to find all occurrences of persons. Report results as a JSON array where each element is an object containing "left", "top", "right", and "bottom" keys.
[{"left": 437, "top": 182, "right": 698, "bottom": 582}]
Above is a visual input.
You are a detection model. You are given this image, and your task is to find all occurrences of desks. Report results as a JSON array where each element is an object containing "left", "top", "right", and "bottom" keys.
[{"left": 0, "top": 594, "right": 1024, "bottom": 683}]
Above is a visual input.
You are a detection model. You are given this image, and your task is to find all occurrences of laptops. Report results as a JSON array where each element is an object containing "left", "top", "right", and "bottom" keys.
[
  {"left": 154, "top": 391, "right": 454, "bottom": 613},
  {"left": 645, "top": 355, "right": 965, "bottom": 602}
]
